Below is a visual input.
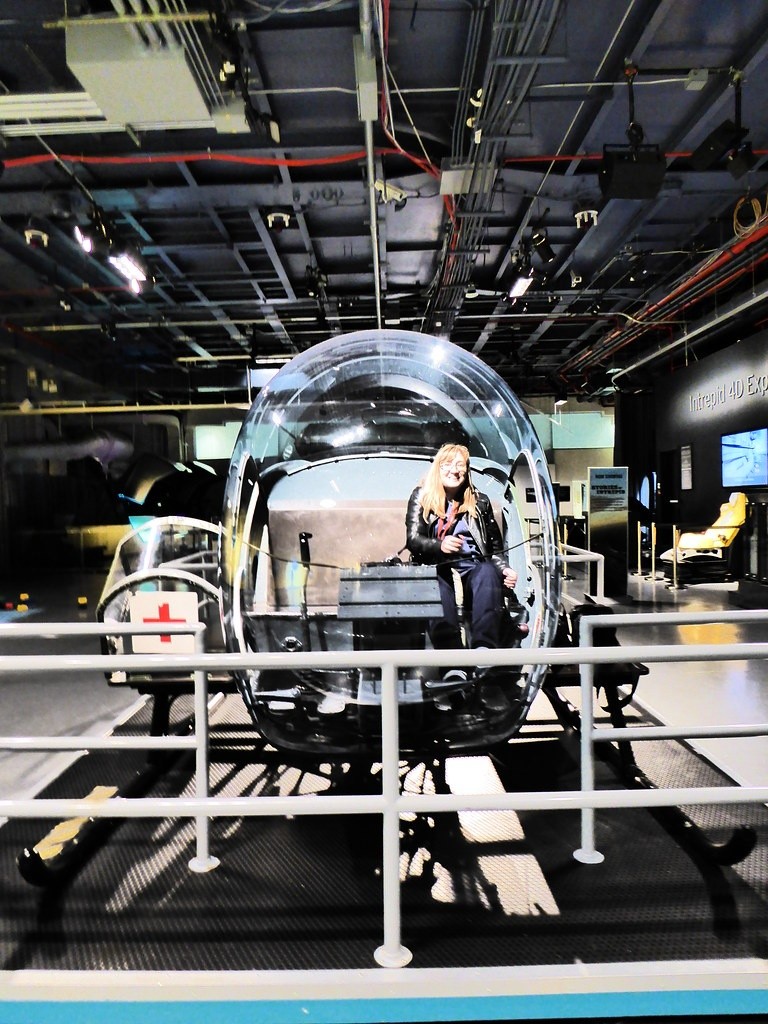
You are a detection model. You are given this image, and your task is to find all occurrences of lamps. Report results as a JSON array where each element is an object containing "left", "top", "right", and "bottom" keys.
[
  {"left": 555, "top": 382, "right": 567, "bottom": 406},
  {"left": 508, "top": 277, "right": 535, "bottom": 298},
  {"left": 531, "top": 266, "right": 548, "bottom": 285},
  {"left": 730, "top": 144, "right": 759, "bottom": 181},
  {"left": 533, "top": 234, "right": 557, "bottom": 263},
  {"left": 599, "top": 77, "right": 665, "bottom": 200},
  {"left": 513, "top": 261, "right": 534, "bottom": 276},
  {"left": 300, "top": 264, "right": 322, "bottom": 298},
  {"left": 693, "top": 77, "right": 750, "bottom": 172}
]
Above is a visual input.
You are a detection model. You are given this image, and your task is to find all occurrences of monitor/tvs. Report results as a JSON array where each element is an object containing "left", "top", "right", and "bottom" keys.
[
  {"left": 720, "top": 425, "right": 767, "bottom": 491},
  {"left": 656, "top": 447, "right": 693, "bottom": 490}
]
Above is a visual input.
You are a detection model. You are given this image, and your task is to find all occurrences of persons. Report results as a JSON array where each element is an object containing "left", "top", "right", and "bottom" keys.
[{"left": 405, "top": 444, "right": 517, "bottom": 712}]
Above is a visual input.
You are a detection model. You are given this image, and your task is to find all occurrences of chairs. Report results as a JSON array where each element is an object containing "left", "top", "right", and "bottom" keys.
[{"left": 678, "top": 492, "right": 749, "bottom": 551}]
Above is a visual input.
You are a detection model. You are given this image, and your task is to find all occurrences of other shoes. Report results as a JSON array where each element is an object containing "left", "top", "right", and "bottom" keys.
[{"left": 442, "top": 669, "right": 467, "bottom": 682}]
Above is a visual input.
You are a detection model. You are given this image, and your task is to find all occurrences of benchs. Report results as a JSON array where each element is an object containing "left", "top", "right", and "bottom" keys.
[{"left": 251, "top": 497, "right": 530, "bottom": 670}]
